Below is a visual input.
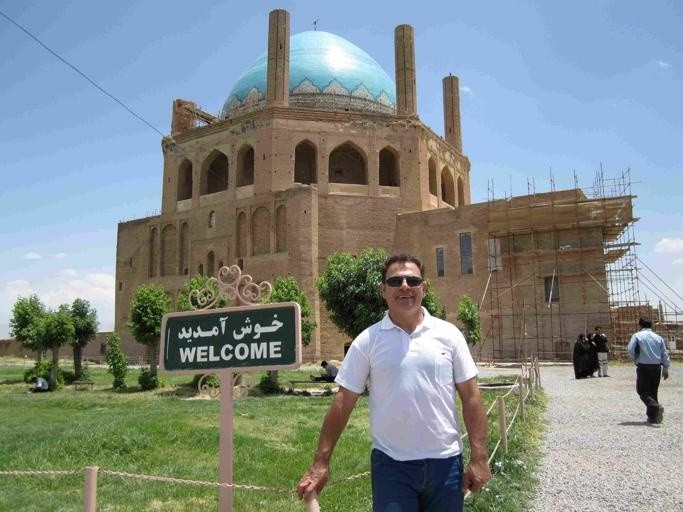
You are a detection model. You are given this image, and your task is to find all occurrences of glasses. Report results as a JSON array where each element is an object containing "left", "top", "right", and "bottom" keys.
[{"left": 385, "top": 275, "right": 425, "bottom": 288}]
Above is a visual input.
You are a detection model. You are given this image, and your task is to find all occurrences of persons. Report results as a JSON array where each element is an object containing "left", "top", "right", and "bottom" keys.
[
  {"left": 297, "top": 254, "right": 493, "bottom": 511},
  {"left": 626, "top": 315, "right": 670, "bottom": 424},
  {"left": 310, "top": 360, "right": 339, "bottom": 382},
  {"left": 573, "top": 327, "right": 611, "bottom": 379},
  {"left": 28, "top": 375, "right": 50, "bottom": 393}
]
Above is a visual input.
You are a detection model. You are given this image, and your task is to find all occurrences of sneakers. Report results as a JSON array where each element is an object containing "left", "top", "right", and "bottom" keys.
[{"left": 645, "top": 403, "right": 664, "bottom": 424}]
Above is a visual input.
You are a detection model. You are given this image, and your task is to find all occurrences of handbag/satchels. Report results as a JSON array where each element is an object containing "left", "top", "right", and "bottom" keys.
[{"left": 634, "top": 343, "right": 640, "bottom": 356}]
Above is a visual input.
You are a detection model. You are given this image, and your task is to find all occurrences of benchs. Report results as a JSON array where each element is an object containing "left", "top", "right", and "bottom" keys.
[
  {"left": 72, "top": 380, "right": 95, "bottom": 392},
  {"left": 289, "top": 381, "right": 340, "bottom": 395}
]
